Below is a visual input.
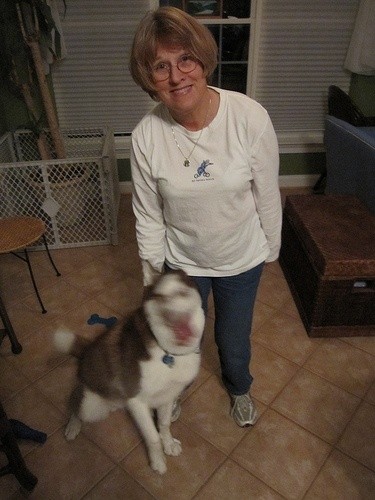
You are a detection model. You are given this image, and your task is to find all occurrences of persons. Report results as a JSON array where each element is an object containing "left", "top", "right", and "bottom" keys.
[{"left": 129, "top": 7, "right": 283, "bottom": 427}]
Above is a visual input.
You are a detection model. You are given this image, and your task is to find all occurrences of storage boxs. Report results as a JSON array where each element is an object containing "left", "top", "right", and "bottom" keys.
[{"left": 278, "top": 192, "right": 375, "bottom": 338}]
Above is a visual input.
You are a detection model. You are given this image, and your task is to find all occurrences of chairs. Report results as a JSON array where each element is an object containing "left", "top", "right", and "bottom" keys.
[{"left": 311, "top": 85, "right": 375, "bottom": 211}]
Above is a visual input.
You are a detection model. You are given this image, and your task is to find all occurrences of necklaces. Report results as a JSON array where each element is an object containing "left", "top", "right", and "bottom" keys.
[{"left": 168, "top": 88, "right": 212, "bottom": 168}]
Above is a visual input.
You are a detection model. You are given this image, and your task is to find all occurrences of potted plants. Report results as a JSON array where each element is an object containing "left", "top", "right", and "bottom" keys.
[{"left": 16, "top": 112, "right": 92, "bottom": 227}]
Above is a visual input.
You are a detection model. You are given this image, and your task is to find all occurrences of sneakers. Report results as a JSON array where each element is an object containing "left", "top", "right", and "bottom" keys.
[
  {"left": 169, "top": 393, "right": 182, "bottom": 422},
  {"left": 229, "top": 390, "right": 256, "bottom": 428}
]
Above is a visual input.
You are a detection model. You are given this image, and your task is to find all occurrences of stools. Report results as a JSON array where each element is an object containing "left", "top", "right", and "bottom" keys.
[{"left": 0, "top": 217, "right": 62, "bottom": 314}]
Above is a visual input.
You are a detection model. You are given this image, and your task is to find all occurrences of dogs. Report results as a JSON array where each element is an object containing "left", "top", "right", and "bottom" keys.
[{"left": 47, "top": 267, "right": 205, "bottom": 475}]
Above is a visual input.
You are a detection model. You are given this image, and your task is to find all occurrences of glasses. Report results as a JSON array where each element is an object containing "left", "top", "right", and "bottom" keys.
[{"left": 149, "top": 51, "right": 199, "bottom": 82}]
